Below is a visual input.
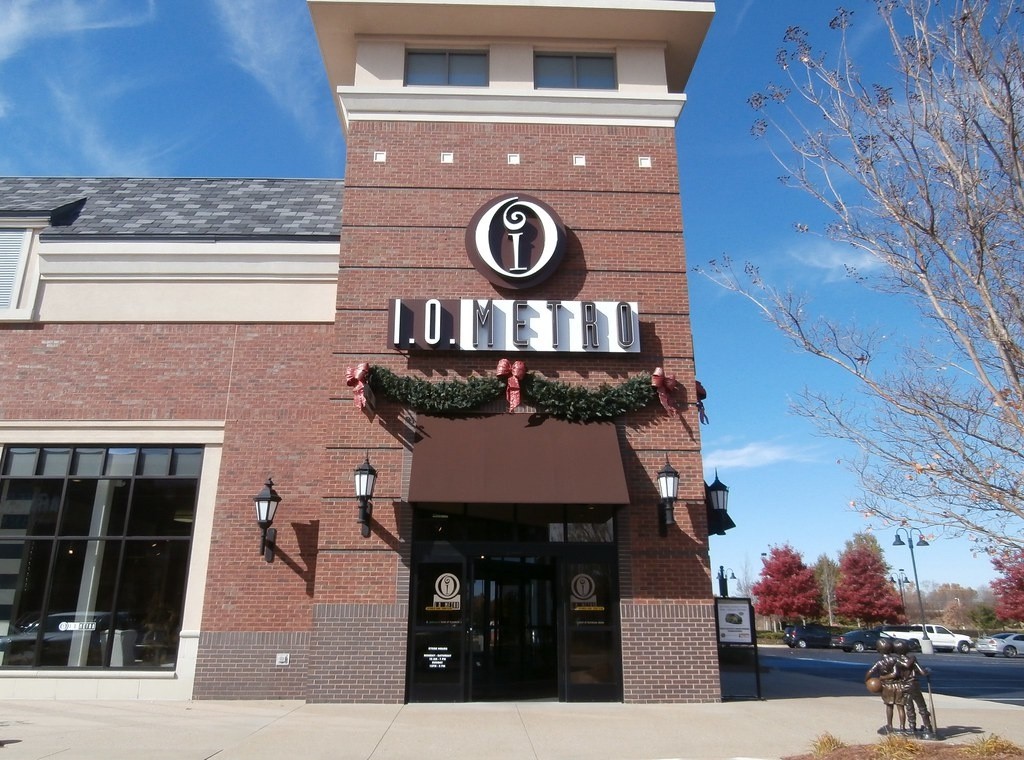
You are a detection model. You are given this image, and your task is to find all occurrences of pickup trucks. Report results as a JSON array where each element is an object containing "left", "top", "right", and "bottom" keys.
[{"left": 877, "top": 624, "right": 974, "bottom": 654}]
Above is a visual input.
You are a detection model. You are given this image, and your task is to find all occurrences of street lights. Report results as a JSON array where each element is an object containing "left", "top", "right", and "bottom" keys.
[
  {"left": 715, "top": 567, "right": 737, "bottom": 596},
  {"left": 889, "top": 573, "right": 911, "bottom": 626},
  {"left": 892, "top": 527, "right": 930, "bottom": 639},
  {"left": 954, "top": 597, "right": 966, "bottom": 630}
]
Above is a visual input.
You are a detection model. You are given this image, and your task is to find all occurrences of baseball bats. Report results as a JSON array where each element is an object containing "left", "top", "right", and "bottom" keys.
[{"left": 927, "top": 675, "right": 937, "bottom": 736}]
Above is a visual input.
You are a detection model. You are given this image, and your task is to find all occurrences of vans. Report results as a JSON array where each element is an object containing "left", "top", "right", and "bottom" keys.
[{"left": 782, "top": 624, "right": 831, "bottom": 649}]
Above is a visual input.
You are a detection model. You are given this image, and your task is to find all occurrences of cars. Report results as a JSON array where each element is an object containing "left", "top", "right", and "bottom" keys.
[
  {"left": 0, "top": 612, "right": 136, "bottom": 665},
  {"left": 833, "top": 630, "right": 880, "bottom": 653},
  {"left": 975, "top": 633, "right": 1024, "bottom": 658}
]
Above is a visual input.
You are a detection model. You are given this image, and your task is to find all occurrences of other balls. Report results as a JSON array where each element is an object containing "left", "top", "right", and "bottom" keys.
[{"left": 865, "top": 677, "right": 882, "bottom": 693}]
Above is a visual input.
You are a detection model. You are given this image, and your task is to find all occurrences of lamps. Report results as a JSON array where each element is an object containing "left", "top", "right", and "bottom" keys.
[
  {"left": 706, "top": 468, "right": 735, "bottom": 535},
  {"left": 657, "top": 452, "right": 680, "bottom": 539},
  {"left": 353, "top": 451, "right": 379, "bottom": 537},
  {"left": 253, "top": 477, "right": 282, "bottom": 564}
]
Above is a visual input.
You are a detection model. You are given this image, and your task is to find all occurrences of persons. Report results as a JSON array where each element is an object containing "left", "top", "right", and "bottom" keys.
[{"left": 865, "top": 639, "right": 933, "bottom": 734}]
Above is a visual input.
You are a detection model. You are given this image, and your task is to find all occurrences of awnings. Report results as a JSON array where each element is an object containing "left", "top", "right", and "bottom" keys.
[{"left": 407, "top": 412, "right": 631, "bottom": 505}]
